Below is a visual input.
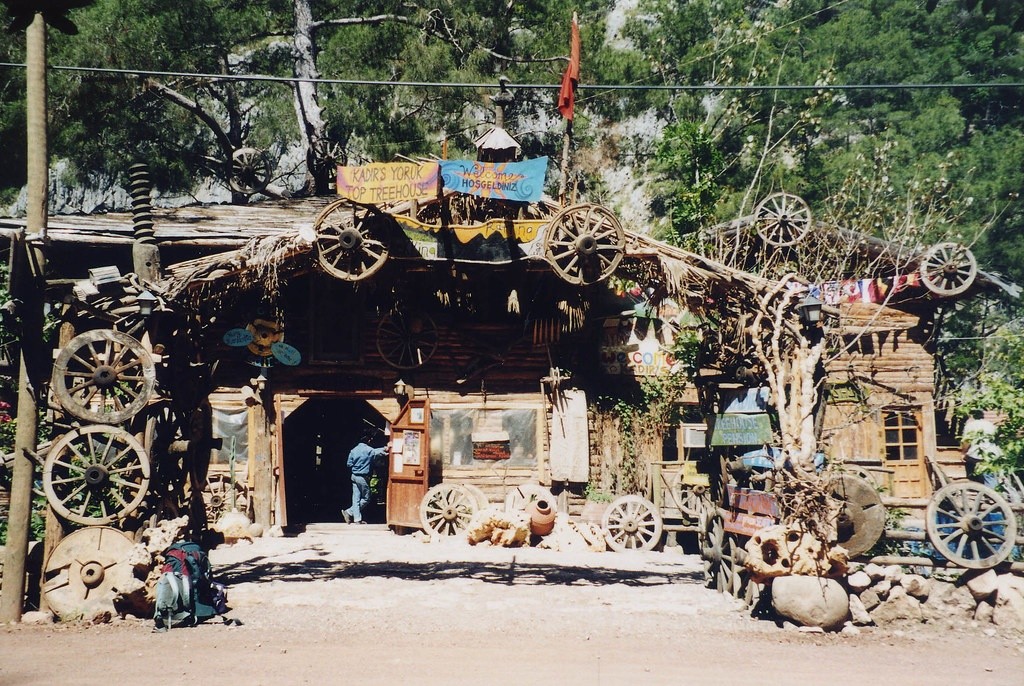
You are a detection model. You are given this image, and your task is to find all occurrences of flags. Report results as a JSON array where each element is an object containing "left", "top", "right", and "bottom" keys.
[{"left": 558, "top": 17, "right": 582, "bottom": 118}]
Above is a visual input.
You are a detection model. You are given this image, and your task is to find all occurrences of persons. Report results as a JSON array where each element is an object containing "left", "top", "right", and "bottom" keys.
[
  {"left": 341, "top": 433, "right": 394, "bottom": 525},
  {"left": 962, "top": 409, "right": 999, "bottom": 491}
]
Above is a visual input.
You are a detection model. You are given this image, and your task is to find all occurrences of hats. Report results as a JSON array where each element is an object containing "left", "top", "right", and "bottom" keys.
[{"left": 245, "top": 318, "right": 284, "bottom": 356}]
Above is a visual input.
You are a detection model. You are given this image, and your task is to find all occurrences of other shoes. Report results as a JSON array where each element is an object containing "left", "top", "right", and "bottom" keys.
[{"left": 342, "top": 510, "right": 351, "bottom": 524}]
